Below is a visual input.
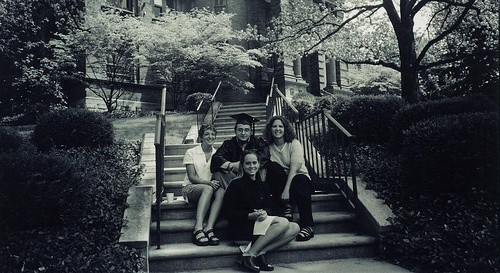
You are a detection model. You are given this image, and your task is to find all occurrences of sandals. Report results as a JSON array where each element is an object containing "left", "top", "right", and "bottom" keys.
[
  {"left": 282, "top": 204, "right": 293, "bottom": 221},
  {"left": 296, "top": 227, "right": 314, "bottom": 241}
]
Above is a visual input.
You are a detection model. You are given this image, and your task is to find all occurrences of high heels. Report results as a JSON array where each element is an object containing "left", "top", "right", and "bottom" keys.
[
  {"left": 192, "top": 230, "right": 209, "bottom": 246},
  {"left": 203, "top": 229, "right": 219, "bottom": 245}
]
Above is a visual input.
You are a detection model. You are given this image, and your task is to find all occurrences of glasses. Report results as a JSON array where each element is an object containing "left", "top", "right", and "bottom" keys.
[{"left": 236, "top": 129, "right": 251, "bottom": 132}]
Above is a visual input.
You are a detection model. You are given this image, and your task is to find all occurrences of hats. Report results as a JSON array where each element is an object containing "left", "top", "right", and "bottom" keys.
[{"left": 229, "top": 113, "right": 259, "bottom": 140}]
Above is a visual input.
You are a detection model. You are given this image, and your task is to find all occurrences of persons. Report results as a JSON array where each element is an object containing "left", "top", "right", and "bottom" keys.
[
  {"left": 182, "top": 123, "right": 227, "bottom": 246},
  {"left": 224, "top": 150, "right": 300, "bottom": 273},
  {"left": 210, "top": 113, "right": 270, "bottom": 191},
  {"left": 262, "top": 115, "right": 314, "bottom": 241}
]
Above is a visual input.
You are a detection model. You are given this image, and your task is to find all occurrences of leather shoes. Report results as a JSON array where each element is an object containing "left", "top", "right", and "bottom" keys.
[
  {"left": 236, "top": 253, "right": 260, "bottom": 273},
  {"left": 253, "top": 255, "right": 274, "bottom": 271}
]
